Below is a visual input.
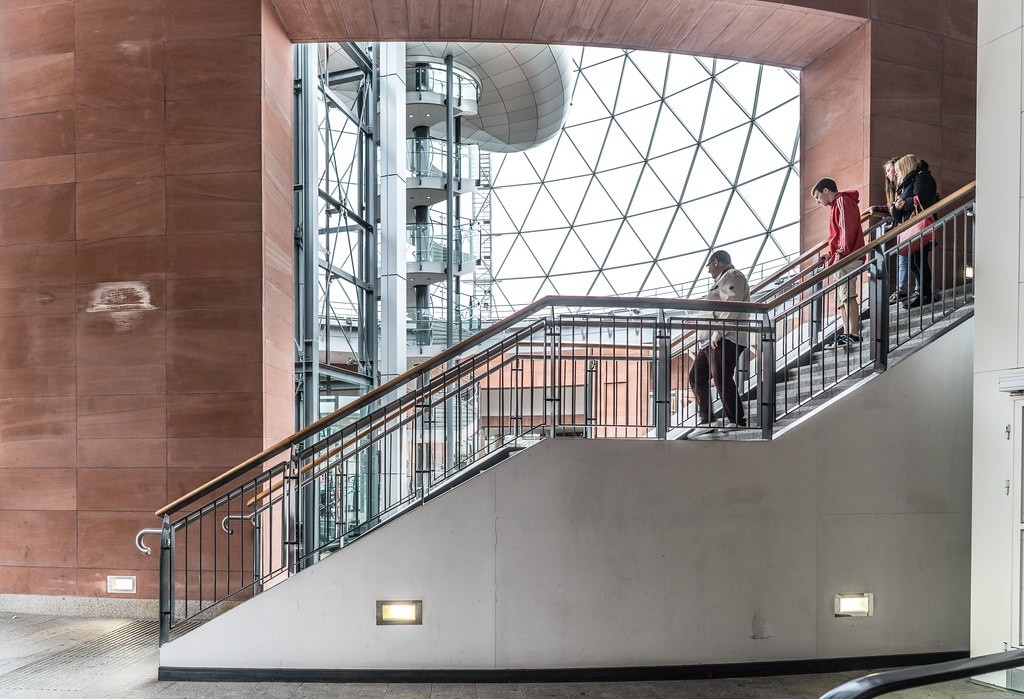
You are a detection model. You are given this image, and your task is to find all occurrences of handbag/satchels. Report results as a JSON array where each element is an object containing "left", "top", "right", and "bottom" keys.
[{"left": 896, "top": 197, "right": 936, "bottom": 256}]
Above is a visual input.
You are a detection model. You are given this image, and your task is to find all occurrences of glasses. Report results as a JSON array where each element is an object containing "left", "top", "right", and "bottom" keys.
[{"left": 706, "top": 258, "right": 719, "bottom": 269}]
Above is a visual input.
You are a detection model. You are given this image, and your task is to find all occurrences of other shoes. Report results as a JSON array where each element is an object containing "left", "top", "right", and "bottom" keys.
[
  {"left": 889, "top": 290, "right": 907, "bottom": 305},
  {"left": 903, "top": 291, "right": 919, "bottom": 306},
  {"left": 911, "top": 295, "right": 937, "bottom": 308}
]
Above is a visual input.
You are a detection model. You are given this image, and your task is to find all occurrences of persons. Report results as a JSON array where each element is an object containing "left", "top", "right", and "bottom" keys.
[
  {"left": 883, "top": 153, "right": 941, "bottom": 310},
  {"left": 811, "top": 177, "right": 867, "bottom": 349},
  {"left": 687, "top": 250, "right": 751, "bottom": 438}
]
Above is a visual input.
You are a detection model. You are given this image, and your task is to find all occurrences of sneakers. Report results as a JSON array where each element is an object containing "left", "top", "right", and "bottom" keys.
[
  {"left": 687, "top": 421, "right": 718, "bottom": 437},
  {"left": 718, "top": 423, "right": 747, "bottom": 432},
  {"left": 823, "top": 335, "right": 849, "bottom": 348},
  {"left": 833, "top": 334, "right": 863, "bottom": 349}
]
